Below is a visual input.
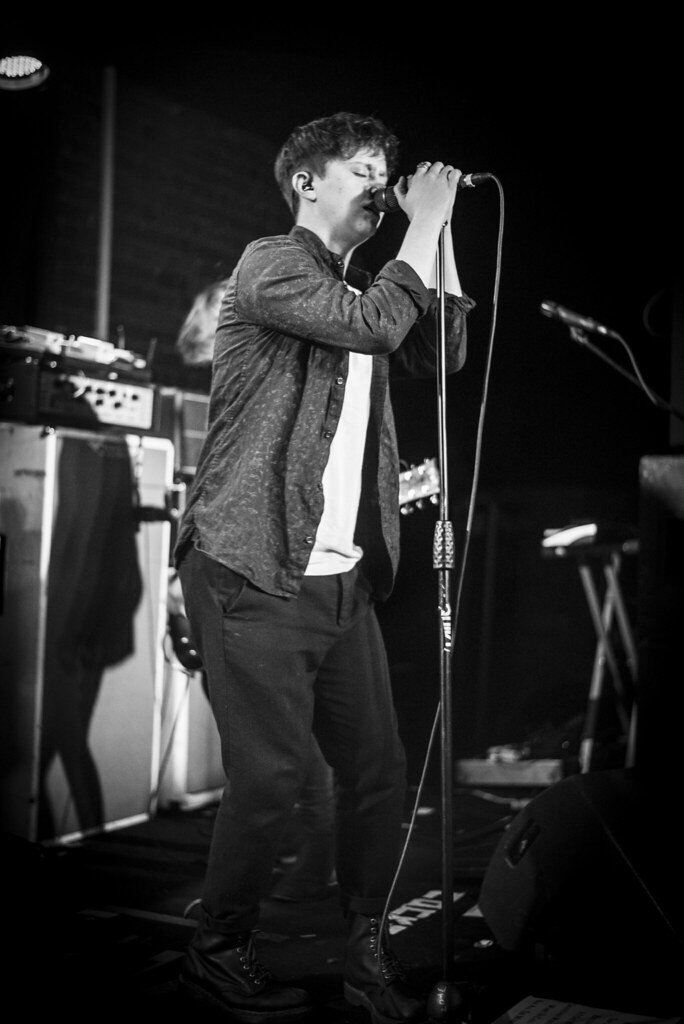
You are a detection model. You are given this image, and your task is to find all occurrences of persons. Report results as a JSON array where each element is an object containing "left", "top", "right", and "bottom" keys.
[{"left": 168, "top": 104, "right": 482, "bottom": 1021}]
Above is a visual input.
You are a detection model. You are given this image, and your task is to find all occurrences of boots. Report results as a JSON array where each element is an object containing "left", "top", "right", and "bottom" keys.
[
  {"left": 342, "top": 912, "right": 431, "bottom": 1023},
  {"left": 176, "top": 919, "right": 316, "bottom": 1019}
]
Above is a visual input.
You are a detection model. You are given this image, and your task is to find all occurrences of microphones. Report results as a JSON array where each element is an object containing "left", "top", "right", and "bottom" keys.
[
  {"left": 373, "top": 173, "right": 493, "bottom": 213},
  {"left": 540, "top": 298, "right": 613, "bottom": 338}
]
